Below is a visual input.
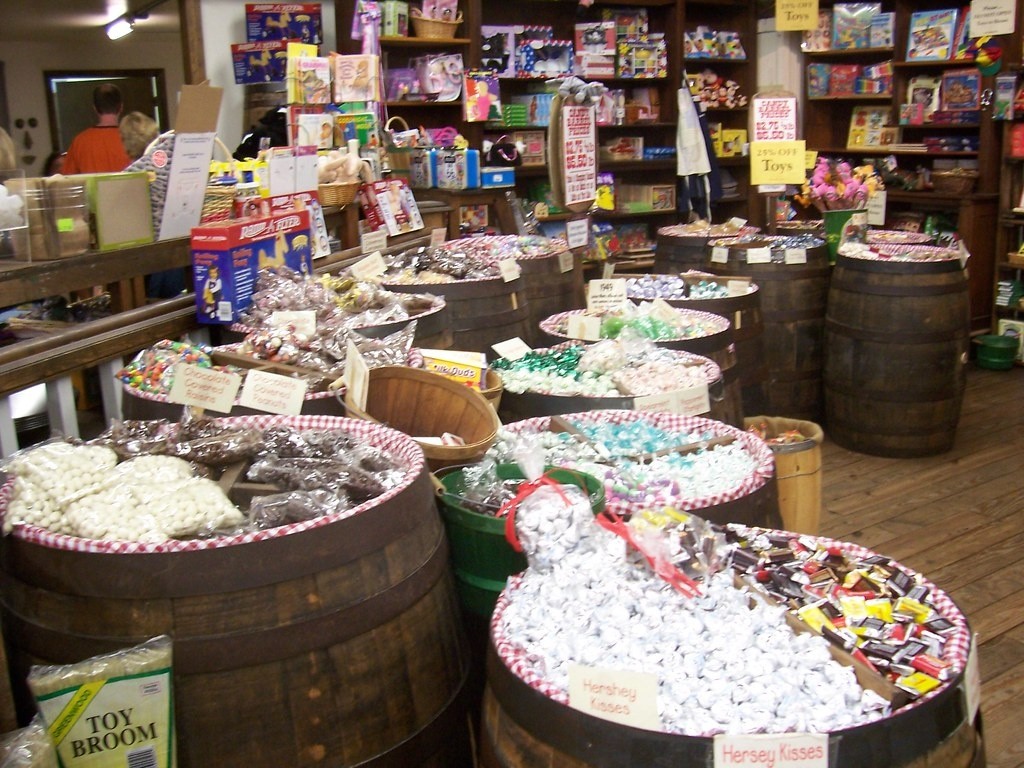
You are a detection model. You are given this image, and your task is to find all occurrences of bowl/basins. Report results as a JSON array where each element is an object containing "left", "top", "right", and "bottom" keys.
[{"left": 975, "top": 336, "right": 1018, "bottom": 370}]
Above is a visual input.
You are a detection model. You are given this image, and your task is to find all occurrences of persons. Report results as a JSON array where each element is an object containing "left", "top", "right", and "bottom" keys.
[
  {"left": 44, "top": 83, "right": 231, "bottom": 299},
  {"left": 699, "top": 68, "right": 747, "bottom": 108}
]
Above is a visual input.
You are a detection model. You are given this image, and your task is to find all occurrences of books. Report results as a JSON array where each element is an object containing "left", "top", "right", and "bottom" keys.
[{"left": 285, "top": 42, "right": 379, "bottom": 150}]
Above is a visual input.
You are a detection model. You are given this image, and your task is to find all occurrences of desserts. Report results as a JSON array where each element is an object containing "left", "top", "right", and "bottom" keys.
[{"left": 808, "top": 158, "right": 867, "bottom": 209}]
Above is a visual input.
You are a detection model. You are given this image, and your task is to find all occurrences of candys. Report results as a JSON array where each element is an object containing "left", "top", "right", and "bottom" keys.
[{"left": 0, "top": 232, "right": 953, "bottom": 733}]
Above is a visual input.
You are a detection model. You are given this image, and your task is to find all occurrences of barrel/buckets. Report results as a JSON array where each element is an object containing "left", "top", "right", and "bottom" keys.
[
  {"left": 656, "top": 222, "right": 826, "bottom": 418},
  {"left": 741, "top": 415, "right": 824, "bottom": 538},
  {"left": 820, "top": 242, "right": 972, "bottom": 460},
  {"left": 402, "top": 352, "right": 503, "bottom": 413},
  {"left": 537, "top": 305, "right": 742, "bottom": 413},
  {"left": 0, "top": 415, "right": 470, "bottom": 768},
  {"left": 487, "top": 340, "right": 728, "bottom": 426},
  {"left": 451, "top": 409, "right": 776, "bottom": 532},
  {"left": 482, "top": 525, "right": 973, "bottom": 768},
  {"left": 425, "top": 461, "right": 606, "bottom": 656},
  {"left": 229, "top": 235, "right": 579, "bottom": 356},
  {"left": 602, "top": 275, "right": 763, "bottom": 393},
  {"left": 329, "top": 364, "right": 499, "bottom": 468},
  {"left": 120, "top": 342, "right": 409, "bottom": 423}
]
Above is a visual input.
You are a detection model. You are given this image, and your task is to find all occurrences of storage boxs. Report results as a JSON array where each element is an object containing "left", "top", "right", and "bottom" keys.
[
  {"left": 3, "top": 179, "right": 89, "bottom": 261},
  {"left": 332, "top": 1, "right": 749, "bottom": 262},
  {"left": 802, "top": 2, "right": 1024, "bottom": 156},
  {"left": 62, "top": 172, "right": 154, "bottom": 253},
  {"left": 122, "top": 0, "right": 335, "bottom": 327}
]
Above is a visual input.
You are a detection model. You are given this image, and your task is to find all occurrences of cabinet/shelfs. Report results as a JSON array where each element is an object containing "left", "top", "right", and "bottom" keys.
[
  {"left": 333, "top": 0, "right": 757, "bottom": 243},
  {"left": 802, "top": 0, "right": 1006, "bottom": 362},
  {"left": 991, "top": 0, "right": 1024, "bottom": 367}
]
